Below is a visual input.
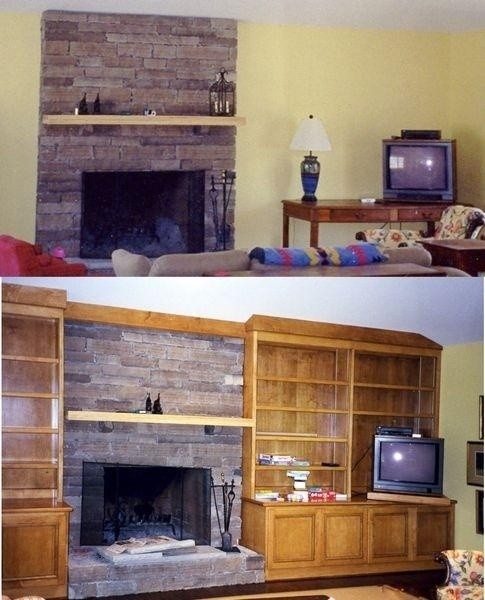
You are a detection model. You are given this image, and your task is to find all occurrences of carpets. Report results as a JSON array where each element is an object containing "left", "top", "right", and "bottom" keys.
[{"left": 195, "top": 583, "right": 427, "bottom": 600}]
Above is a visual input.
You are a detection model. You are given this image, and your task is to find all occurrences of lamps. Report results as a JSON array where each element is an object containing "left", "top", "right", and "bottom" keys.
[{"left": 288, "top": 115, "right": 332, "bottom": 201}]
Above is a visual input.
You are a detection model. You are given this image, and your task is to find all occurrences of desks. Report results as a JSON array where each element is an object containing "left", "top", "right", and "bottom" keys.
[{"left": 280, "top": 198, "right": 473, "bottom": 248}]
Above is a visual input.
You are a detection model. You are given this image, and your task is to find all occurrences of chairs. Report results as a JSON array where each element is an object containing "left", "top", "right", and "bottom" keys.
[
  {"left": 432, "top": 548, "right": 484, "bottom": 600},
  {"left": 355, "top": 204, "right": 485, "bottom": 248},
  {"left": 1, "top": 235, "right": 87, "bottom": 277}
]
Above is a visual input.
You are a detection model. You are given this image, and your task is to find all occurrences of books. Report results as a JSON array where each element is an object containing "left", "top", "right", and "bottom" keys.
[{"left": 97, "top": 534, "right": 196, "bottom": 565}]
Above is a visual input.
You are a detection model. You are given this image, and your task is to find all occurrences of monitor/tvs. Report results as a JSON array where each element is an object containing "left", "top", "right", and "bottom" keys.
[
  {"left": 381, "top": 138, "right": 458, "bottom": 202},
  {"left": 373, "top": 433, "right": 444, "bottom": 496}
]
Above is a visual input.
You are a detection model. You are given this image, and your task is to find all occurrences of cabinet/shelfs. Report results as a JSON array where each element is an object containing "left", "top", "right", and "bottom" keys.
[
  {"left": 2, "top": 284, "right": 73, "bottom": 600},
  {"left": 242, "top": 330, "right": 457, "bottom": 580}
]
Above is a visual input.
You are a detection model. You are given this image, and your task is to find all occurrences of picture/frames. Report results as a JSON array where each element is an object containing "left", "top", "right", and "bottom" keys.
[
  {"left": 476, "top": 489, "right": 483, "bottom": 535},
  {"left": 467, "top": 442, "right": 484, "bottom": 487},
  {"left": 478, "top": 395, "right": 484, "bottom": 441}
]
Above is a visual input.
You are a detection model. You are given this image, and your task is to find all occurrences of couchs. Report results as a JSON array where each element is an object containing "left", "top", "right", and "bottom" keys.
[{"left": 110, "top": 238, "right": 474, "bottom": 277}]
